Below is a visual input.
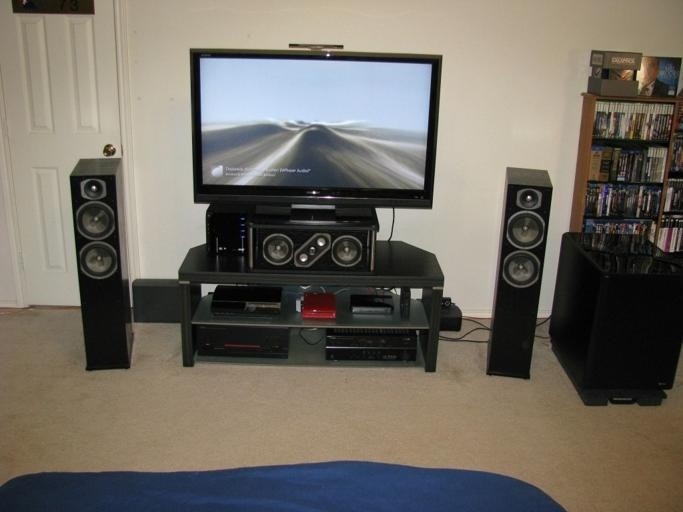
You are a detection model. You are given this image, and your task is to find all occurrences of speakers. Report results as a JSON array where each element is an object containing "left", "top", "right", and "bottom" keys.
[
  {"left": 69, "top": 157, "right": 131, "bottom": 370},
  {"left": 249, "top": 224, "right": 376, "bottom": 274},
  {"left": 485, "top": 166, "right": 554, "bottom": 379}
]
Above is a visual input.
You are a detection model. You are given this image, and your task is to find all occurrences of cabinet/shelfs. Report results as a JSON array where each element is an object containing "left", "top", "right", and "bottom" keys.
[
  {"left": 568, "top": 91, "right": 681, "bottom": 254},
  {"left": 178, "top": 239, "right": 443, "bottom": 373}
]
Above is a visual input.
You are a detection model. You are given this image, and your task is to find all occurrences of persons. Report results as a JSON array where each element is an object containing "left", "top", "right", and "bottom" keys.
[
  {"left": 636, "top": 57, "right": 674, "bottom": 96},
  {"left": 578, "top": 103, "right": 683, "bottom": 274}
]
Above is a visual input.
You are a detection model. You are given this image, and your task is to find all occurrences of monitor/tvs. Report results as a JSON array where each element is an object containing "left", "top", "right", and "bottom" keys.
[{"left": 189, "top": 48, "right": 443, "bottom": 225}]
